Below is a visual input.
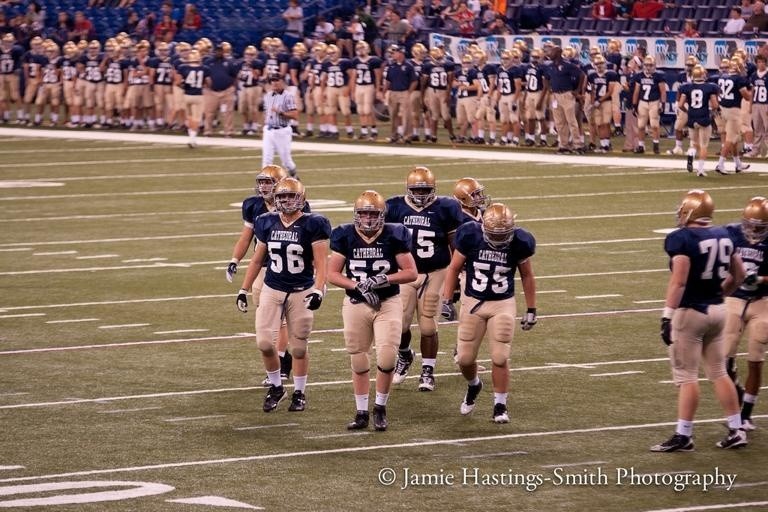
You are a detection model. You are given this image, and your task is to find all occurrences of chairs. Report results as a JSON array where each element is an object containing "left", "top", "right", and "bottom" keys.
[
  {"left": 518, "top": 0, "right": 768, "bottom": 39},
  {"left": 7, "top": 0, "right": 341, "bottom": 56}
]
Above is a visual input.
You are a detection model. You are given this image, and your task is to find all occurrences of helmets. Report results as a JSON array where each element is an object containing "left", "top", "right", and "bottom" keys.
[
  {"left": 743, "top": 196, "right": 768, "bottom": 241},
  {"left": 353, "top": 190, "right": 386, "bottom": 233},
  {"left": 3, "top": 34, "right": 764, "bottom": 78},
  {"left": 675, "top": 190, "right": 713, "bottom": 227},
  {"left": 406, "top": 167, "right": 437, "bottom": 206},
  {"left": 453, "top": 178, "right": 491, "bottom": 208},
  {"left": 275, "top": 178, "right": 306, "bottom": 215},
  {"left": 256, "top": 166, "right": 286, "bottom": 200},
  {"left": 482, "top": 203, "right": 515, "bottom": 247}
]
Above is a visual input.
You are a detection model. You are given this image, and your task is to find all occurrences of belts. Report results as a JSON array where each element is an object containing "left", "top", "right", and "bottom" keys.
[{"left": 273, "top": 125, "right": 287, "bottom": 129}]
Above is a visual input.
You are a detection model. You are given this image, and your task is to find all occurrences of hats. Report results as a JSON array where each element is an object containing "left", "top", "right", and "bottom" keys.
[{"left": 271, "top": 73, "right": 283, "bottom": 80}]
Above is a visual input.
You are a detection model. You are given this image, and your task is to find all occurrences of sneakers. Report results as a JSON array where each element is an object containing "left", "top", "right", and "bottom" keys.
[
  {"left": 449, "top": 135, "right": 691, "bottom": 155},
  {"left": 460, "top": 380, "right": 483, "bottom": 415},
  {"left": 492, "top": 403, "right": 510, "bottom": 423},
  {"left": 716, "top": 384, "right": 756, "bottom": 448},
  {"left": 418, "top": 365, "right": 436, "bottom": 391},
  {"left": 261, "top": 349, "right": 292, "bottom": 386},
  {"left": 373, "top": 402, "right": 388, "bottom": 430},
  {"left": 288, "top": 391, "right": 306, "bottom": 411},
  {"left": 347, "top": 410, "right": 369, "bottom": 430},
  {"left": 687, "top": 147, "right": 768, "bottom": 177},
  {"left": 263, "top": 385, "right": 287, "bottom": 412},
  {"left": 392, "top": 349, "right": 415, "bottom": 386},
  {"left": 294, "top": 131, "right": 438, "bottom": 145},
  {"left": 0, "top": 120, "right": 265, "bottom": 132},
  {"left": 189, "top": 140, "right": 197, "bottom": 148},
  {"left": 650, "top": 432, "right": 694, "bottom": 452}
]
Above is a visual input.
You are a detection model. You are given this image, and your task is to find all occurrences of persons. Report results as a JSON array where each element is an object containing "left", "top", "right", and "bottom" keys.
[
  {"left": 225, "top": 164, "right": 311, "bottom": 385},
  {"left": 326, "top": 190, "right": 419, "bottom": 431},
  {"left": 1, "top": 0, "right": 768, "bottom": 182},
  {"left": 649, "top": 189, "right": 749, "bottom": 453},
  {"left": 235, "top": 178, "right": 332, "bottom": 411},
  {"left": 448, "top": 178, "right": 491, "bottom": 305},
  {"left": 440, "top": 203, "right": 539, "bottom": 423},
  {"left": 726, "top": 197, "right": 768, "bottom": 432},
  {"left": 385, "top": 166, "right": 463, "bottom": 392}
]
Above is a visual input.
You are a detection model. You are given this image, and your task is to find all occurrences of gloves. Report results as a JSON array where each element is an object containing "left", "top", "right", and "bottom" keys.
[
  {"left": 355, "top": 274, "right": 390, "bottom": 311},
  {"left": 744, "top": 270, "right": 757, "bottom": 286},
  {"left": 225, "top": 262, "right": 238, "bottom": 283},
  {"left": 236, "top": 289, "right": 248, "bottom": 314},
  {"left": 661, "top": 318, "right": 674, "bottom": 346},
  {"left": 594, "top": 100, "right": 601, "bottom": 109},
  {"left": 658, "top": 103, "right": 665, "bottom": 113},
  {"left": 520, "top": 308, "right": 537, "bottom": 330},
  {"left": 512, "top": 102, "right": 517, "bottom": 110},
  {"left": 441, "top": 278, "right": 461, "bottom": 321},
  {"left": 632, "top": 104, "right": 639, "bottom": 117},
  {"left": 304, "top": 290, "right": 323, "bottom": 311}
]
